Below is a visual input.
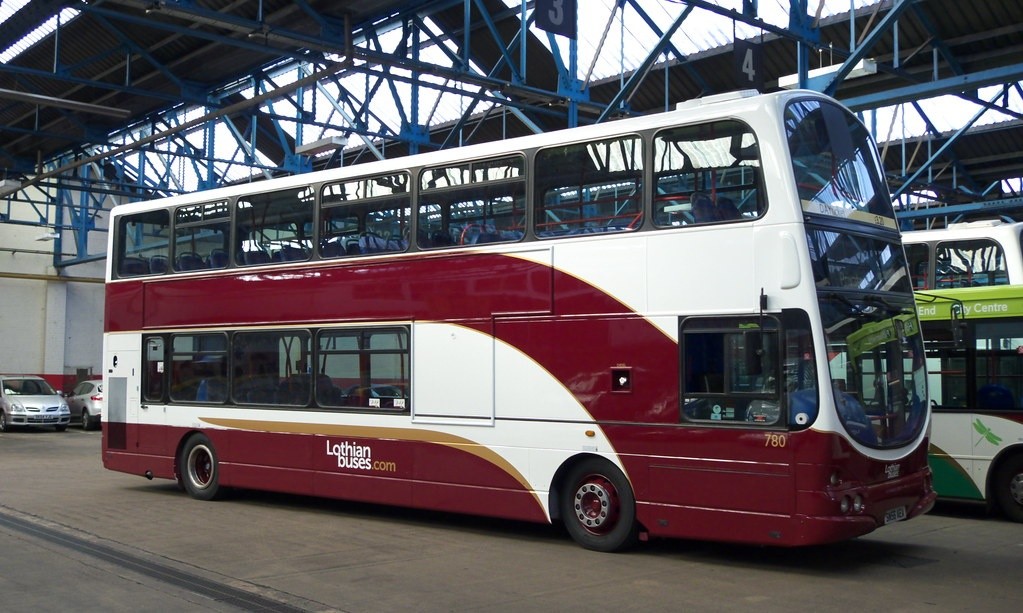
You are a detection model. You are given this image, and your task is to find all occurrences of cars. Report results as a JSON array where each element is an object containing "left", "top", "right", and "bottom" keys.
[
  {"left": 0, "top": 375, "right": 70, "bottom": 432},
  {"left": 66, "top": 380, "right": 102, "bottom": 430}
]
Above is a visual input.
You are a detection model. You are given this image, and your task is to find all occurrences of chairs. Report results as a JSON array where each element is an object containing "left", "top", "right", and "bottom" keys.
[{"left": 116, "top": 189, "right": 1015, "bottom": 409}]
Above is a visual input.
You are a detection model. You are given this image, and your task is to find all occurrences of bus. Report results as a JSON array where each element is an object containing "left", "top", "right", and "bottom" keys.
[
  {"left": 892, "top": 216, "right": 1023, "bottom": 525},
  {"left": 101, "top": 90, "right": 968, "bottom": 553}
]
